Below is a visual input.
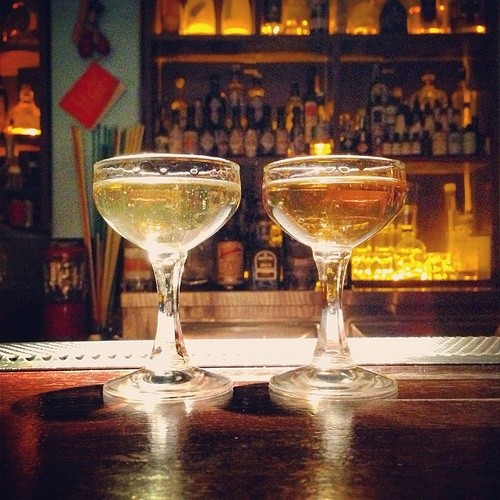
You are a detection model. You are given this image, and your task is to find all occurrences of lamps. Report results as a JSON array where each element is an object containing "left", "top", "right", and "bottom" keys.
[{"left": 6, "top": 84, "right": 42, "bottom": 136}]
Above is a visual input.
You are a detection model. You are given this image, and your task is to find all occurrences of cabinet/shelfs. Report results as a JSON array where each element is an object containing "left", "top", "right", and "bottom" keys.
[{"left": 120, "top": 0, "right": 500, "bottom": 338}]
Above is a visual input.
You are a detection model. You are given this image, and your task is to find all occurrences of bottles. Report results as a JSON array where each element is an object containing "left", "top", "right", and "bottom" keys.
[
  {"left": 122, "top": 1, "right": 482, "bottom": 292},
  {"left": 0, "top": 129, "right": 34, "bottom": 228}
]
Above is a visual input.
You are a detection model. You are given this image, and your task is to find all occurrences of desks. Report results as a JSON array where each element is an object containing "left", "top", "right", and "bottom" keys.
[{"left": 1, "top": 338, "right": 500, "bottom": 500}]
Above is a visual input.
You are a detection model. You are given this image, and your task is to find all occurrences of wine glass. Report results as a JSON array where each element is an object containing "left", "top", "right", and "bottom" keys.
[
  {"left": 263, "top": 156, "right": 409, "bottom": 401},
  {"left": 93, "top": 152, "right": 241, "bottom": 405}
]
